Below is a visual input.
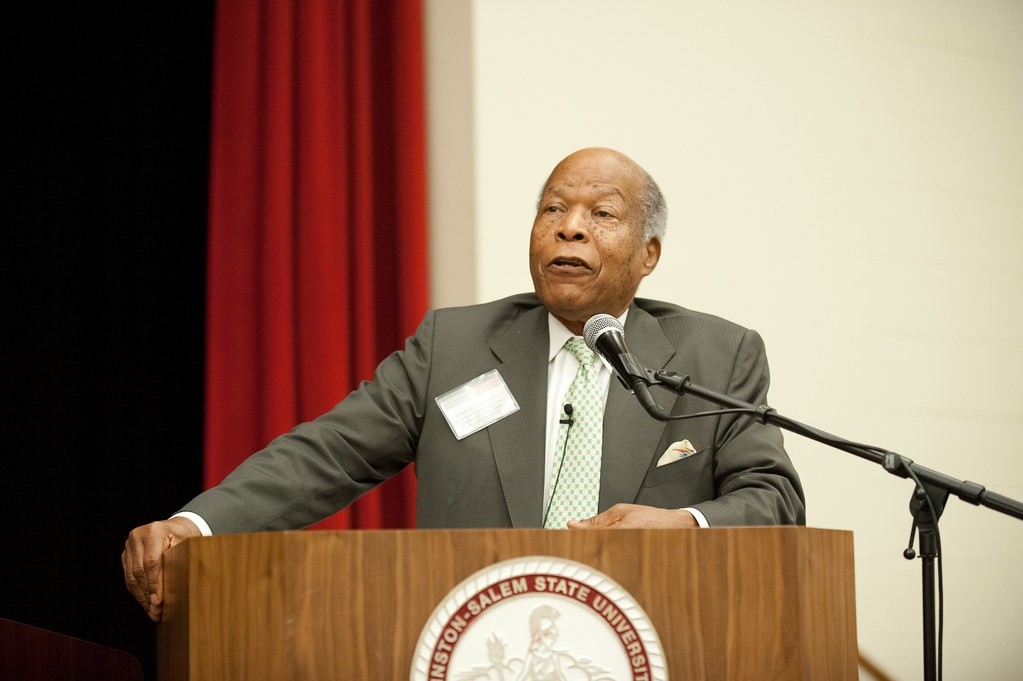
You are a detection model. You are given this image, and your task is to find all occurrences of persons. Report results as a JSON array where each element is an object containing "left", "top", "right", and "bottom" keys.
[{"left": 121, "top": 148, "right": 806, "bottom": 621}]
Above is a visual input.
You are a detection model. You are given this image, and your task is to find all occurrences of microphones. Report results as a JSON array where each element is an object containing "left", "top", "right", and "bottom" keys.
[
  {"left": 583, "top": 314, "right": 667, "bottom": 421},
  {"left": 560, "top": 403, "right": 574, "bottom": 425}
]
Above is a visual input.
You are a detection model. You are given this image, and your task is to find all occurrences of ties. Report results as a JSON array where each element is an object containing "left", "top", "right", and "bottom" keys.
[{"left": 542, "top": 339, "right": 605, "bottom": 529}]
